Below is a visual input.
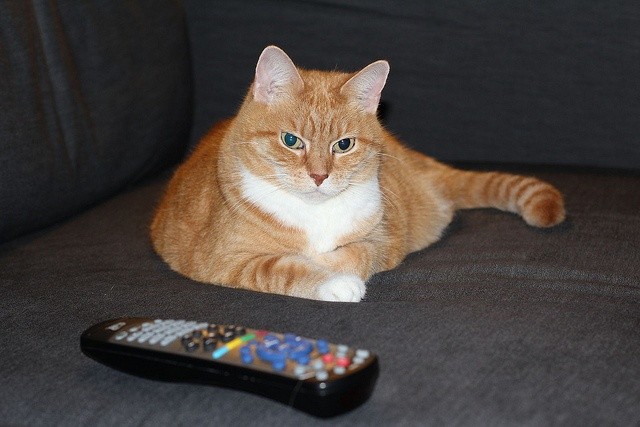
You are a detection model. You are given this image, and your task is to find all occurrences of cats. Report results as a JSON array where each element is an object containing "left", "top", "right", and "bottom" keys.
[{"left": 149, "top": 45, "right": 567, "bottom": 302}]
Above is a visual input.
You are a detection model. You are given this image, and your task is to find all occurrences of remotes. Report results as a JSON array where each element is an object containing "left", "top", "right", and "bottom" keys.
[{"left": 80, "top": 314, "right": 379, "bottom": 418}]
